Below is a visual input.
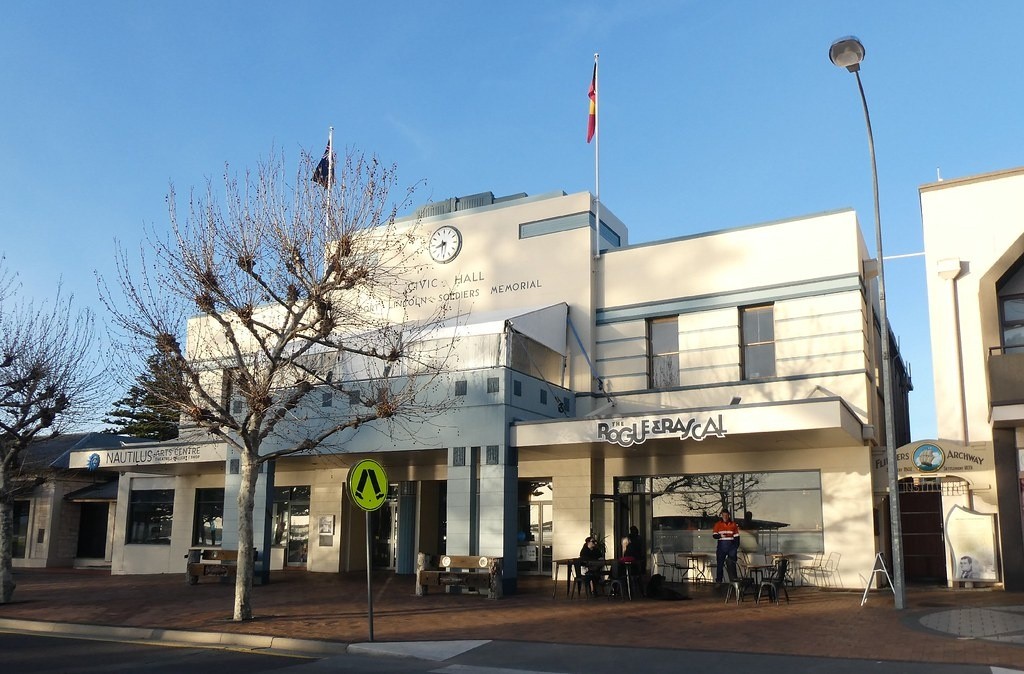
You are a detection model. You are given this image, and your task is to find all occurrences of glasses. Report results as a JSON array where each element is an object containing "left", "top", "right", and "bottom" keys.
[{"left": 589, "top": 541, "right": 595, "bottom": 544}]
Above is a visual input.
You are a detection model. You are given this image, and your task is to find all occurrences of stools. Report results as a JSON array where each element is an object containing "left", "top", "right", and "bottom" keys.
[{"left": 607, "top": 579, "right": 627, "bottom": 601}]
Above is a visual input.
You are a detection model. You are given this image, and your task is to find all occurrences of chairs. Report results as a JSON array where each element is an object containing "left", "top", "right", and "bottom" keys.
[
  {"left": 724, "top": 561, "right": 757, "bottom": 607},
  {"left": 736, "top": 547, "right": 763, "bottom": 595},
  {"left": 708, "top": 557, "right": 726, "bottom": 583},
  {"left": 757, "top": 559, "right": 789, "bottom": 606},
  {"left": 651, "top": 547, "right": 683, "bottom": 583},
  {"left": 674, "top": 551, "right": 706, "bottom": 585},
  {"left": 568, "top": 558, "right": 600, "bottom": 601}
]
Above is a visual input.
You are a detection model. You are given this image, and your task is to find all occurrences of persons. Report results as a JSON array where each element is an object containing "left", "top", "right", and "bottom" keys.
[
  {"left": 713, "top": 509, "right": 740, "bottom": 588},
  {"left": 622, "top": 526, "right": 648, "bottom": 599},
  {"left": 580, "top": 537, "right": 603, "bottom": 598},
  {"left": 960, "top": 555, "right": 974, "bottom": 579}
]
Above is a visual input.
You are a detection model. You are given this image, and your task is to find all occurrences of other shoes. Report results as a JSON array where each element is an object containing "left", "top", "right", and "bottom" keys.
[{"left": 715, "top": 582, "right": 721, "bottom": 587}]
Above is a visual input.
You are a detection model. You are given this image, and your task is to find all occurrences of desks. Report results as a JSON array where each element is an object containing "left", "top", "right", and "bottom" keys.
[
  {"left": 742, "top": 563, "right": 773, "bottom": 601},
  {"left": 270, "top": 546, "right": 287, "bottom": 571},
  {"left": 551, "top": 559, "right": 631, "bottom": 601},
  {"left": 679, "top": 554, "right": 708, "bottom": 585}
]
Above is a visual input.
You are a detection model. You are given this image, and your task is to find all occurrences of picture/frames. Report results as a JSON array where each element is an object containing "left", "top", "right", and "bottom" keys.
[{"left": 318, "top": 514, "right": 335, "bottom": 536}]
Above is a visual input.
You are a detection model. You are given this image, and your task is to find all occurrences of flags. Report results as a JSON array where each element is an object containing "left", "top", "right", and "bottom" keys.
[
  {"left": 587, "top": 64, "right": 596, "bottom": 143},
  {"left": 310, "top": 140, "right": 334, "bottom": 190}
]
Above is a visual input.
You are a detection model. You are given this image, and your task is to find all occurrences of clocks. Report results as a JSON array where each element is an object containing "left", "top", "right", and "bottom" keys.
[{"left": 428, "top": 225, "right": 462, "bottom": 264}]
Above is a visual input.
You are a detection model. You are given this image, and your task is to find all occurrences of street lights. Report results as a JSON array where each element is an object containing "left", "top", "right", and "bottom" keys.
[{"left": 827, "top": 37, "right": 908, "bottom": 611}]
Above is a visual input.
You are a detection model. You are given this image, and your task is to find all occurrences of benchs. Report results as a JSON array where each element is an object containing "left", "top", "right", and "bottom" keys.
[
  {"left": 184, "top": 547, "right": 258, "bottom": 589},
  {"left": 415, "top": 552, "right": 504, "bottom": 601}
]
